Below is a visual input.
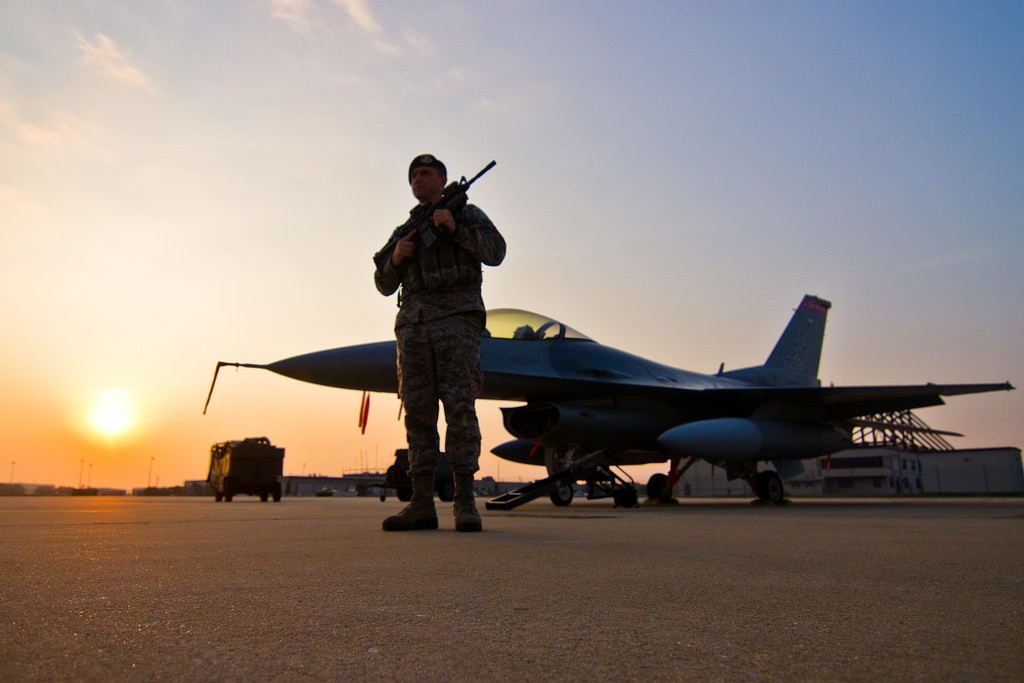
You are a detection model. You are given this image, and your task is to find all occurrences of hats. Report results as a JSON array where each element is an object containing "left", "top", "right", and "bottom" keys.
[{"left": 409, "top": 154, "right": 446, "bottom": 186}]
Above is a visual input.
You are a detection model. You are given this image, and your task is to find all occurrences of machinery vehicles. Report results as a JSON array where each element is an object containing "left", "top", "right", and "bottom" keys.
[{"left": 206, "top": 437, "right": 285, "bottom": 502}]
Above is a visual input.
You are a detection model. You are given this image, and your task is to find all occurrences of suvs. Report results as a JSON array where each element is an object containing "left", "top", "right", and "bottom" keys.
[{"left": 385, "top": 449, "right": 455, "bottom": 503}]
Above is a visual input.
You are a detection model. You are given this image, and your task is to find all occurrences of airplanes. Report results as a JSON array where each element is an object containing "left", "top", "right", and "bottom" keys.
[{"left": 204, "top": 293, "right": 1014, "bottom": 508}]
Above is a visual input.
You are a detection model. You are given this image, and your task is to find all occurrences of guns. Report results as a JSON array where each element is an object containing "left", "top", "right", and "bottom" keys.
[{"left": 371, "top": 158, "right": 498, "bottom": 278}]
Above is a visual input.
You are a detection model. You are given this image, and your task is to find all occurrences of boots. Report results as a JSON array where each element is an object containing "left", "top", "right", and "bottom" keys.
[
  {"left": 382, "top": 478, "right": 439, "bottom": 531},
  {"left": 452, "top": 470, "right": 482, "bottom": 533}
]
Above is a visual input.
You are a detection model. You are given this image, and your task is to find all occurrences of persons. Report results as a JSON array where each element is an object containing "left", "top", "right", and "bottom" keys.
[{"left": 373, "top": 155, "right": 506, "bottom": 529}]
